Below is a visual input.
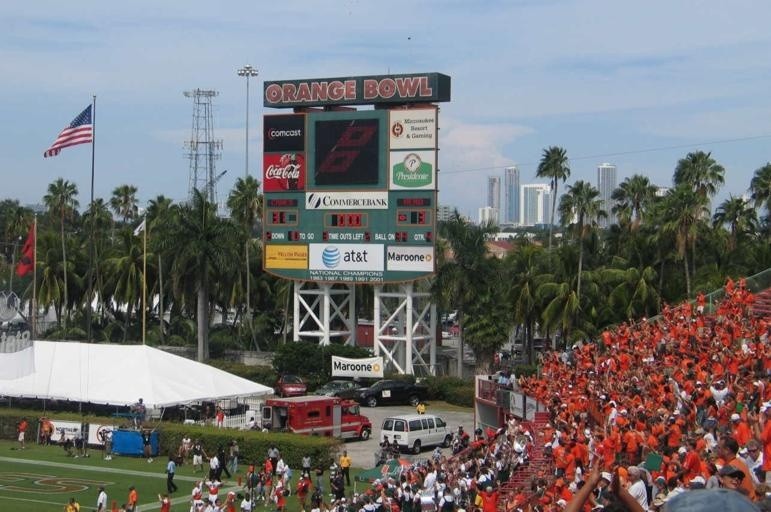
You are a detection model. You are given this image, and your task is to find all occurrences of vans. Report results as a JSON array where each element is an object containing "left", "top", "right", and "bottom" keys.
[{"left": 380, "top": 414, "right": 454, "bottom": 454}]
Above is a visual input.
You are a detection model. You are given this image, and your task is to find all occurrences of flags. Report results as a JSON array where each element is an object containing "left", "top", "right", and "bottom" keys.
[
  {"left": 44, "top": 103, "right": 94, "bottom": 158},
  {"left": 14, "top": 221, "right": 36, "bottom": 278},
  {"left": 132, "top": 219, "right": 145, "bottom": 236}
]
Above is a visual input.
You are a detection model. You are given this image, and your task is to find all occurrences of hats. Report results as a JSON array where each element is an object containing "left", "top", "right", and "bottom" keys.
[
  {"left": 669, "top": 410, "right": 680, "bottom": 423},
  {"left": 656, "top": 477, "right": 665, "bottom": 484},
  {"left": 599, "top": 472, "right": 612, "bottom": 483},
  {"left": 679, "top": 447, "right": 687, "bottom": 454},
  {"left": 689, "top": 448, "right": 765, "bottom": 485},
  {"left": 729, "top": 414, "right": 740, "bottom": 421},
  {"left": 556, "top": 499, "right": 566, "bottom": 511},
  {"left": 599, "top": 395, "right": 627, "bottom": 415}
]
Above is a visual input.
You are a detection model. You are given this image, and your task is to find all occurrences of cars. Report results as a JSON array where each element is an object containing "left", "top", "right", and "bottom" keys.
[
  {"left": 313, "top": 380, "right": 429, "bottom": 407},
  {"left": 513, "top": 339, "right": 548, "bottom": 353},
  {"left": 275, "top": 375, "right": 307, "bottom": 398}
]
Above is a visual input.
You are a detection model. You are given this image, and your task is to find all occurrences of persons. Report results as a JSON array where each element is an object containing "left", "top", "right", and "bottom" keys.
[
  {"left": 18, "top": 396, "right": 353, "bottom": 511},
  {"left": 340, "top": 276, "right": 771, "bottom": 510}
]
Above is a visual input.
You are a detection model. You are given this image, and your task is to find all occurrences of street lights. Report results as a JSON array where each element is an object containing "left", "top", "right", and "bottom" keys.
[{"left": 237, "top": 64, "right": 259, "bottom": 178}]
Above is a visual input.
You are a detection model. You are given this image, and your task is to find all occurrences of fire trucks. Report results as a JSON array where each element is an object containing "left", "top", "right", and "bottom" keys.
[{"left": 262, "top": 394, "right": 371, "bottom": 441}]
